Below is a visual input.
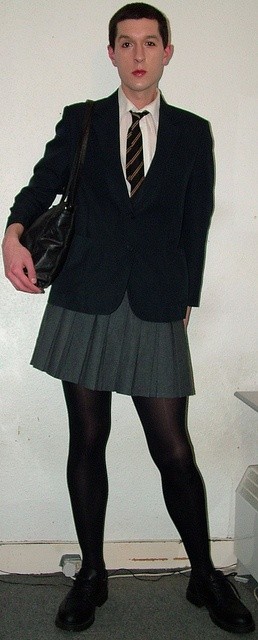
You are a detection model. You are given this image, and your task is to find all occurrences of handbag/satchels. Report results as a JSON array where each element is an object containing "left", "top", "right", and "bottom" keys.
[{"left": 16, "top": 100, "right": 94, "bottom": 290}]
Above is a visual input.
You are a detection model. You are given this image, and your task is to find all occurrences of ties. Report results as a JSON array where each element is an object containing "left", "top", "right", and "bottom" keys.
[{"left": 127, "top": 110, "right": 149, "bottom": 196}]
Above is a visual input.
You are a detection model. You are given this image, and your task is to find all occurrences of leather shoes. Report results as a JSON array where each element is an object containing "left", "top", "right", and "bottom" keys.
[
  {"left": 55, "top": 565, "right": 108, "bottom": 632},
  {"left": 186, "top": 569, "right": 255, "bottom": 633}
]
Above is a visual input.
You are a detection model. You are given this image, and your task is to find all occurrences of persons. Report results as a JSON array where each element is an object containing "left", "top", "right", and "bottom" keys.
[{"left": 0, "top": 1, "right": 258, "bottom": 636}]
